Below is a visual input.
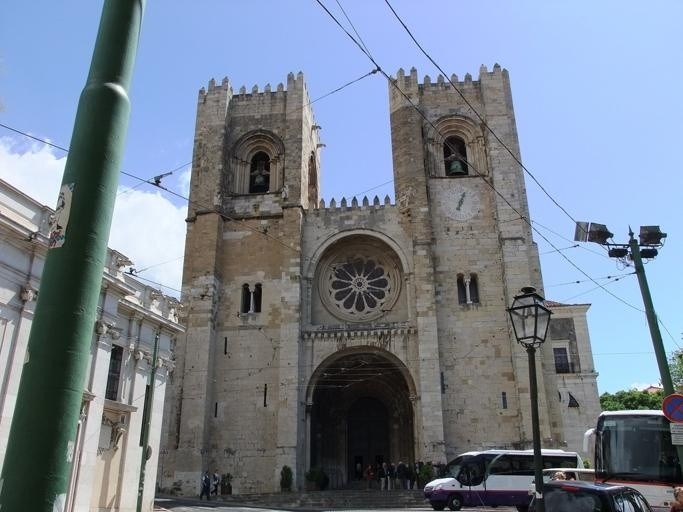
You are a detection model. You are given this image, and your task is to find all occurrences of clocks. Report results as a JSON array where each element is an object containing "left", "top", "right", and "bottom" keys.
[{"left": 441, "top": 186, "right": 481, "bottom": 221}]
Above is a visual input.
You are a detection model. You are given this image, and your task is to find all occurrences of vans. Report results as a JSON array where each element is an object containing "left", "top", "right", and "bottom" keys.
[{"left": 528, "top": 468, "right": 595, "bottom": 496}]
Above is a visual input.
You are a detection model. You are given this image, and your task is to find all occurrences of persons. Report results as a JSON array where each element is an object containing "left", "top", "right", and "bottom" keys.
[
  {"left": 553, "top": 472, "right": 566, "bottom": 482},
  {"left": 353, "top": 460, "right": 361, "bottom": 481},
  {"left": 565, "top": 472, "right": 575, "bottom": 482},
  {"left": 362, "top": 461, "right": 447, "bottom": 492},
  {"left": 197, "top": 471, "right": 210, "bottom": 502},
  {"left": 316, "top": 467, "right": 325, "bottom": 491},
  {"left": 667, "top": 486, "right": 683, "bottom": 512},
  {"left": 209, "top": 469, "right": 221, "bottom": 497}
]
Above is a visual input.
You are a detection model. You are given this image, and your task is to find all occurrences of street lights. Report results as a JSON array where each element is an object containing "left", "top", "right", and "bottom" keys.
[{"left": 505, "top": 287, "right": 554, "bottom": 512}]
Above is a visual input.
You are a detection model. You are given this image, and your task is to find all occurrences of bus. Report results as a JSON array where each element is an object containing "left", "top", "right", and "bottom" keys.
[
  {"left": 582, "top": 409, "right": 683, "bottom": 512},
  {"left": 424, "top": 448, "right": 585, "bottom": 512}
]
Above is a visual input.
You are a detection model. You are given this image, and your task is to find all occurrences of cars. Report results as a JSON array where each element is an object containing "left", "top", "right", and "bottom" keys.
[{"left": 528, "top": 480, "right": 654, "bottom": 512}]
